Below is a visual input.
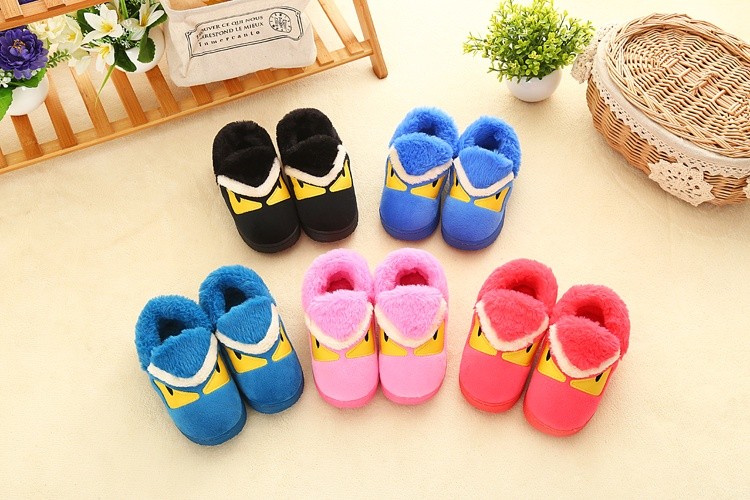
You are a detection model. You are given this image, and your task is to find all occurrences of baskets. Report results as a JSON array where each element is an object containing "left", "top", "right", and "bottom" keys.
[{"left": 573, "top": 12, "right": 749, "bottom": 208}]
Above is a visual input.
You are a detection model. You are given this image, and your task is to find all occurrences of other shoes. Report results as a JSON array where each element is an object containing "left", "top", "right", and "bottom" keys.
[
  {"left": 302, "top": 246, "right": 378, "bottom": 407},
  {"left": 458, "top": 259, "right": 558, "bottom": 413},
  {"left": 441, "top": 114, "right": 521, "bottom": 250},
  {"left": 134, "top": 294, "right": 245, "bottom": 445},
  {"left": 373, "top": 249, "right": 446, "bottom": 405},
  {"left": 378, "top": 106, "right": 459, "bottom": 241},
  {"left": 524, "top": 284, "right": 631, "bottom": 437},
  {"left": 275, "top": 108, "right": 360, "bottom": 244},
  {"left": 212, "top": 120, "right": 301, "bottom": 251},
  {"left": 200, "top": 266, "right": 303, "bottom": 415}
]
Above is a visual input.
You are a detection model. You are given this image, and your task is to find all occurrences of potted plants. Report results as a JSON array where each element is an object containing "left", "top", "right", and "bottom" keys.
[{"left": 464, "top": 1, "right": 597, "bottom": 102}]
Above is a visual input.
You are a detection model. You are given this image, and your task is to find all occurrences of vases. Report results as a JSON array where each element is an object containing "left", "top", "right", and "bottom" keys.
[
  {"left": 6, "top": 72, "right": 51, "bottom": 117},
  {"left": 103, "top": 25, "right": 164, "bottom": 74}
]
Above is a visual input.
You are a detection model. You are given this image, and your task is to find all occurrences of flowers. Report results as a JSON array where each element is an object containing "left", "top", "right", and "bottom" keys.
[
  {"left": 65, "top": 0, "right": 168, "bottom": 107},
  {"left": 1, "top": 16, "right": 81, "bottom": 117}
]
